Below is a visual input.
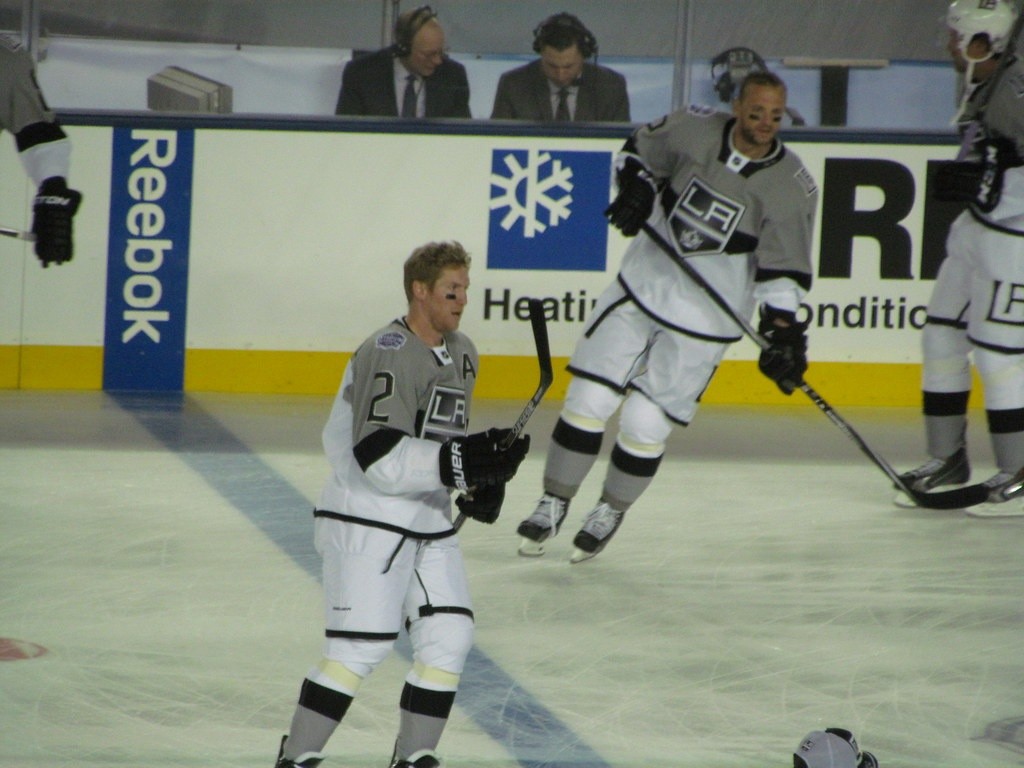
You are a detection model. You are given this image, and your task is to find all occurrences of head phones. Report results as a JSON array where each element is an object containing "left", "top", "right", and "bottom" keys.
[
  {"left": 711, "top": 46, "right": 770, "bottom": 101},
  {"left": 533, "top": 15, "right": 596, "bottom": 59},
  {"left": 392, "top": 4, "right": 437, "bottom": 57}
]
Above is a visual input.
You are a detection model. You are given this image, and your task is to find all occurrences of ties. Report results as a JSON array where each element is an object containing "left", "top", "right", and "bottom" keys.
[
  {"left": 554, "top": 89, "right": 573, "bottom": 122},
  {"left": 400, "top": 74, "right": 418, "bottom": 119}
]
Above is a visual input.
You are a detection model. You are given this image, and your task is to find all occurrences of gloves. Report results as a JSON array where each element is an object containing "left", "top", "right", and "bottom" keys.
[
  {"left": 603, "top": 155, "right": 661, "bottom": 237},
  {"left": 439, "top": 427, "right": 531, "bottom": 491},
  {"left": 31, "top": 175, "right": 83, "bottom": 270},
  {"left": 454, "top": 483, "right": 506, "bottom": 525},
  {"left": 757, "top": 301, "right": 808, "bottom": 396}
]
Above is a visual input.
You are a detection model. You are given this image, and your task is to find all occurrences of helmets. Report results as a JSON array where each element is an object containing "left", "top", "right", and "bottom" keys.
[{"left": 937, "top": 0, "right": 1017, "bottom": 65}]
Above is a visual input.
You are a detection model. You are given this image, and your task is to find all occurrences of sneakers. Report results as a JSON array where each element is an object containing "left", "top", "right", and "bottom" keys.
[
  {"left": 964, "top": 472, "right": 1024, "bottom": 518},
  {"left": 515, "top": 489, "right": 570, "bottom": 558},
  {"left": 274, "top": 734, "right": 326, "bottom": 768},
  {"left": 891, "top": 456, "right": 972, "bottom": 507},
  {"left": 567, "top": 500, "right": 625, "bottom": 564},
  {"left": 386, "top": 738, "right": 442, "bottom": 768}
]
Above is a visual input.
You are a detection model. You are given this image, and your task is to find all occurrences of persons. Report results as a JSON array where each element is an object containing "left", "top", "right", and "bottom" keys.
[
  {"left": 488, "top": 12, "right": 631, "bottom": 123},
  {"left": 275, "top": 238, "right": 530, "bottom": 768},
  {"left": 335, "top": 5, "right": 472, "bottom": 119},
  {"left": 515, "top": 70, "right": 819, "bottom": 564},
  {"left": 1, "top": 33, "right": 83, "bottom": 269},
  {"left": 792, "top": 727, "right": 878, "bottom": 768},
  {"left": 889, "top": 0, "right": 1024, "bottom": 517}
]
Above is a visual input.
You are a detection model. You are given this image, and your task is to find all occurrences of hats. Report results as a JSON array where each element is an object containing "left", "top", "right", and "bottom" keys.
[{"left": 791, "top": 727, "right": 880, "bottom": 768}]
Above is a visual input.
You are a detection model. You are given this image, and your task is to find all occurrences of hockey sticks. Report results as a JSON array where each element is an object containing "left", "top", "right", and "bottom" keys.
[
  {"left": 639, "top": 220, "right": 990, "bottom": 509},
  {"left": 0, "top": 225, "right": 37, "bottom": 242},
  {"left": 451, "top": 298, "right": 555, "bottom": 532}
]
